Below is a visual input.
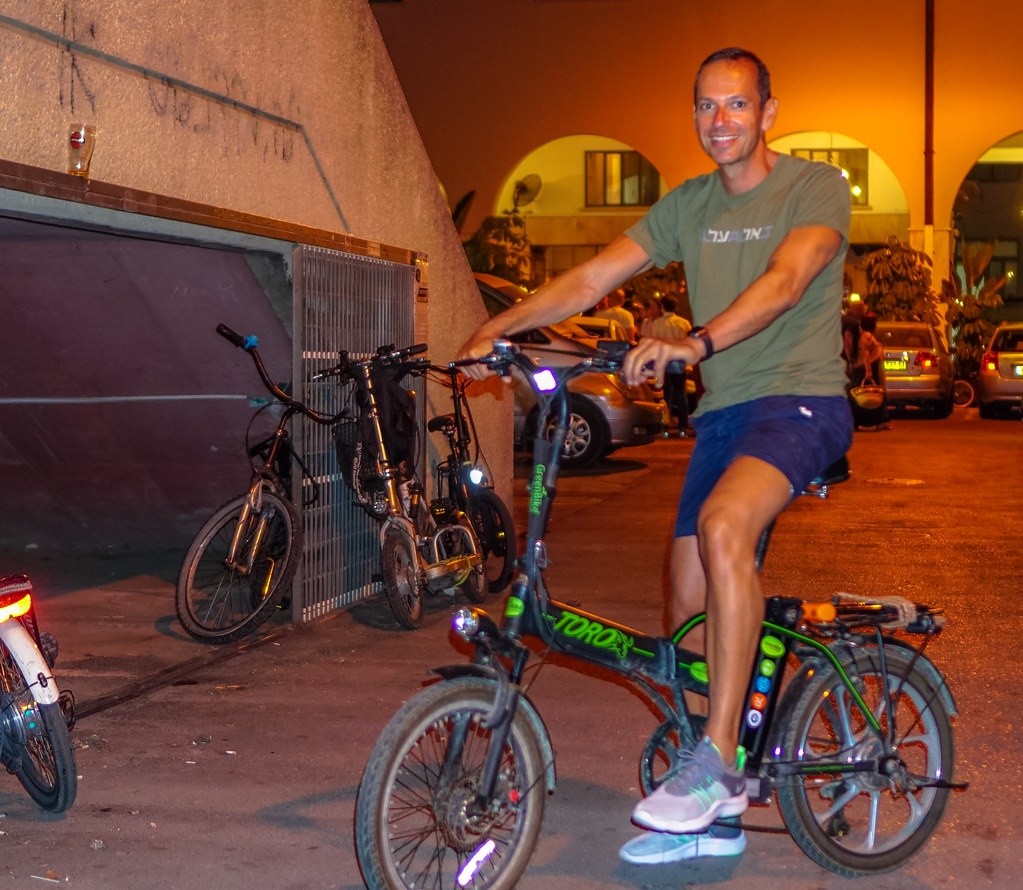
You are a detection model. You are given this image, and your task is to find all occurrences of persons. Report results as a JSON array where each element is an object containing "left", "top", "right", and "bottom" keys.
[
  {"left": 583, "top": 287, "right": 693, "bottom": 439},
  {"left": 456, "top": 48, "right": 855, "bottom": 866},
  {"left": 840, "top": 300, "right": 893, "bottom": 432}
]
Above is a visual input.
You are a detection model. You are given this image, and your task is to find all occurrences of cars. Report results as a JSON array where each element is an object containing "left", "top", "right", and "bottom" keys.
[
  {"left": 871, "top": 319, "right": 958, "bottom": 418},
  {"left": 976, "top": 321, "right": 1023, "bottom": 418},
  {"left": 465, "top": 272, "right": 692, "bottom": 469}
]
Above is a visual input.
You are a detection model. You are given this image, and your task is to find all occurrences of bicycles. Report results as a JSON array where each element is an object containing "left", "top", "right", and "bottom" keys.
[
  {"left": 350, "top": 332, "right": 976, "bottom": 890},
  {"left": 397, "top": 349, "right": 520, "bottom": 592},
  {"left": 173, "top": 325, "right": 359, "bottom": 649},
  {"left": 307, "top": 337, "right": 485, "bottom": 627},
  {"left": 0, "top": 567, "right": 78, "bottom": 815}
]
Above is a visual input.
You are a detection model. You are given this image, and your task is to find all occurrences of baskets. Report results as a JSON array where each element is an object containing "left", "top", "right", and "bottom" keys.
[
  {"left": 851, "top": 377, "right": 884, "bottom": 408},
  {"left": 332, "top": 423, "right": 382, "bottom": 491}
]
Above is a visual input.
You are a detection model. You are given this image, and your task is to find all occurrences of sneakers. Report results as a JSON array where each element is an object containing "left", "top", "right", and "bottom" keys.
[
  {"left": 619, "top": 814, "right": 748, "bottom": 864},
  {"left": 633, "top": 736, "right": 750, "bottom": 833}
]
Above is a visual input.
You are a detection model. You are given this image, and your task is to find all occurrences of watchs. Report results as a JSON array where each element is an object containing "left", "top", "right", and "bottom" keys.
[{"left": 688, "top": 326, "right": 712, "bottom": 361}]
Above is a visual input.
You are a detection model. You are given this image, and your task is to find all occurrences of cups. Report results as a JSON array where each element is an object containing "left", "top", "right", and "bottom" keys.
[{"left": 68, "top": 123, "right": 95, "bottom": 176}]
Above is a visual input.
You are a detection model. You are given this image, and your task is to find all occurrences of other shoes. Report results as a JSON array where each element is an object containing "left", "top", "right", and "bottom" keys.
[{"left": 876, "top": 425, "right": 893, "bottom": 431}]
